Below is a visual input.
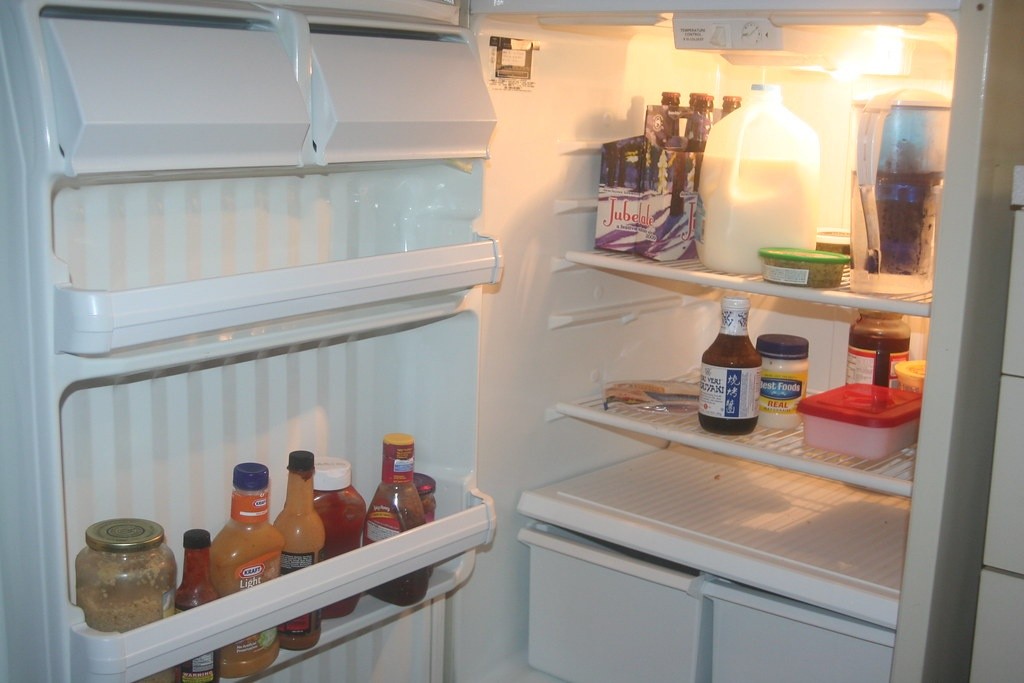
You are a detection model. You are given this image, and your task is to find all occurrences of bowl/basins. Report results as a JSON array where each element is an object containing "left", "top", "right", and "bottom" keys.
[{"left": 759, "top": 245, "right": 850, "bottom": 288}]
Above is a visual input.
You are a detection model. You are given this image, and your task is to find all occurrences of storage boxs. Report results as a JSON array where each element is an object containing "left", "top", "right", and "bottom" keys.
[
  {"left": 794, "top": 381, "right": 922, "bottom": 459},
  {"left": 699, "top": 577, "right": 895, "bottom": 683},
  {"left": 516, "top": 519, "right": 713, "bottom": 683}
]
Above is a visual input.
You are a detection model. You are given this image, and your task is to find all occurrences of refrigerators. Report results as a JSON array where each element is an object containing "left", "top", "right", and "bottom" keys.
[{"left": 0, "top": 0, "right": 1024, "bottom": 683}]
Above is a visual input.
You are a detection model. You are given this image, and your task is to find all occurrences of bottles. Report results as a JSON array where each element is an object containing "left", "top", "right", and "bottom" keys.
[
  {"left": 175, "top": 529, "right": 220, "bottom": 683},
  {"left": 683, "top": 92, "right": 697, "bottom": 152},
  {"left": 699, "top": 296, "right": 763, "bottom": 434},
  {"left": 313, "top": 455, "right": 367, "bottom": 618},
  {"left": 363, "top": 433, "right": 429, "bottom": 606},
  {"left": 659, "top": 91, "right": 681, "bottom": 149},
  {"left": 692, "top": 83, "right": 821, "bottom": 276},
  {"left": 721, "top": 95, "right": 742, "bottom": 118},
  {"left": 209, "top": 462, "right": 285, "bottom": 678},
  {"left": 686, "top": 92, "right": 714, "bottom": 152},
  {"left": 273, "top": 450, "right": 325, "bottom": 650}
]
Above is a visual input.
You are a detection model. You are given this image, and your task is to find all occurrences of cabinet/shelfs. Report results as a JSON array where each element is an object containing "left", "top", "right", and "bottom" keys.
[
  {"left": 550, "top": 247, "right": 931, "bottom": 497},
  {"left": 967, "top": 165, "right": 1024, "bottom": 683}
]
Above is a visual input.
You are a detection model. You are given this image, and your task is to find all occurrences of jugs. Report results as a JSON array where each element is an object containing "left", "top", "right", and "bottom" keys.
[{"left": 846, "top": 89, "right": 952, "bottom": 294}]
